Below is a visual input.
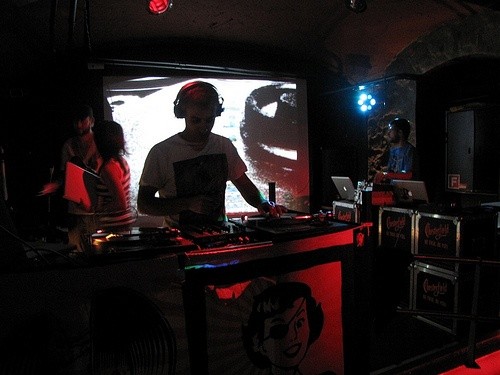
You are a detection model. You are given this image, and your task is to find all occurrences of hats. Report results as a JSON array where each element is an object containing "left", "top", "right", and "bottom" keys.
[{"left": 71, "top": 105, "right": 93, "bottom": 118}]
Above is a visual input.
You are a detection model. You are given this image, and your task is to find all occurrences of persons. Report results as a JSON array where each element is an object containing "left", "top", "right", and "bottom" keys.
[
  {"left": 38, "top": 105, "right": 106, "bottom": 233},
  {"left": 373, "top": 119, "right": 420, "bottom": 192},
  {"left": 0, "top": 146, "right": 21, "bottom": 245},
  {"left": 78, "top": 119, "right": 134, "bottom": 236},
  {"left": 137, "top": 82, "right": 288, "bottom": 228}
]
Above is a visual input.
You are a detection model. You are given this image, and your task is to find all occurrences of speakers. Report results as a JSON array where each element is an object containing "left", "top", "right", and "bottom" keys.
[{"left": 444, "top": 104, "right": 500, "bottom": 195}]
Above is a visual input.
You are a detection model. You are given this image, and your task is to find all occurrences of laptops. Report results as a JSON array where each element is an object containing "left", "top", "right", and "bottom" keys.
[
  {"left": 393, "top": 180, "right": 430, "bottom": 210},
  {"left": 331, "top": 176, "right": 356, "bottom": 200}
]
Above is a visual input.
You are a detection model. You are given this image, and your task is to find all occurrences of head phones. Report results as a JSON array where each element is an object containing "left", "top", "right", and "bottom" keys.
[{"left": 174, "top": 82, "right": 224, "bottom": 118}]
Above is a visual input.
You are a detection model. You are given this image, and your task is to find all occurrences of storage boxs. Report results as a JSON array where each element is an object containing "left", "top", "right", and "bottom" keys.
[
  {"left": 332, "top": 201, "right": 359, "bottom": 226},
  {"left": 378, "top": 206, "right": 414, "bottom": 260},
  {"left": 407, "top": 264, "right": 458, "bottom": 337},
  {"left": 413, "top": 211, "right": 461, "bottom": 279}
]
